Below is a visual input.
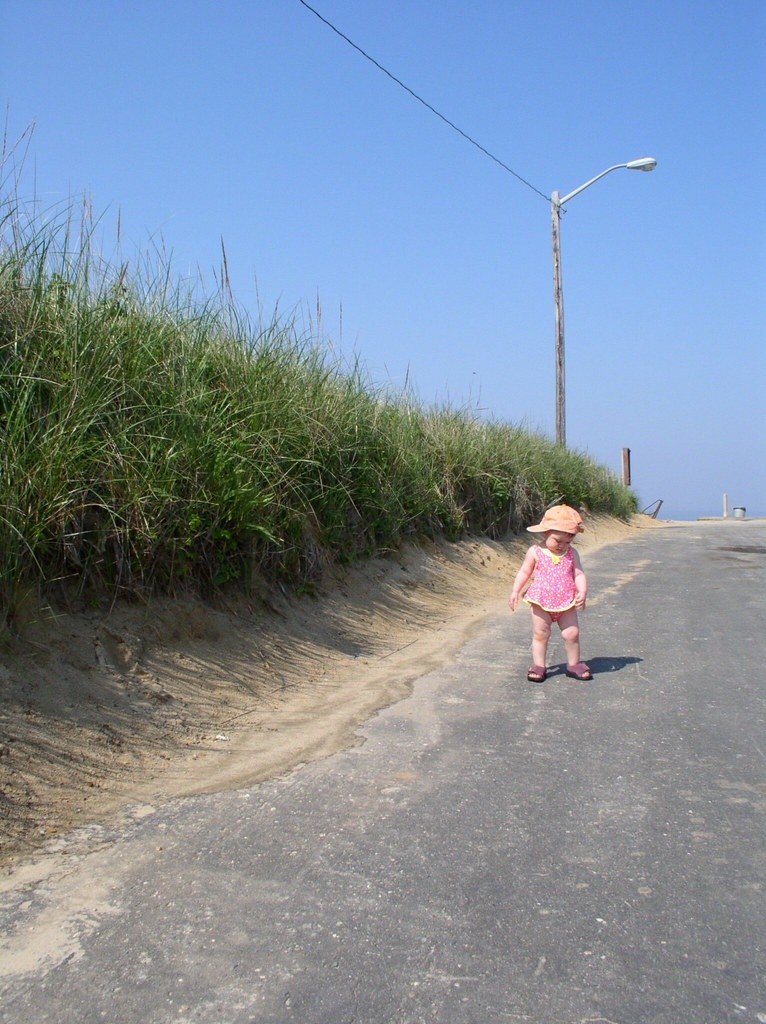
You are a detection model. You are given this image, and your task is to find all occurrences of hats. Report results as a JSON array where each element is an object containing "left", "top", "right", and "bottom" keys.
[{"left": 526, "top": 505, "right": 584, "bottom": 535}]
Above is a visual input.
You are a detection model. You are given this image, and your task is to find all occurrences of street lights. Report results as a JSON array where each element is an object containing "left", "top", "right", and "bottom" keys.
[{"left": 551, "top": 156, "right": 657, "bottom": 449}]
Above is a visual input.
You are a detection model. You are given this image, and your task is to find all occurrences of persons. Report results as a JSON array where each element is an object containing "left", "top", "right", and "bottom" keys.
[{"left": 509, "top": 504, "right": 593, "bottom": 683}]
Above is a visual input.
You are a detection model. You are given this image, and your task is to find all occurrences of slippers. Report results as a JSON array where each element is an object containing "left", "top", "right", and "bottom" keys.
[
  {"left": 566, "top": 660, "right": 593, "bottom": 681},
  {"left": 528, "top": 664, "right": 547, "bottom": 682}
]
